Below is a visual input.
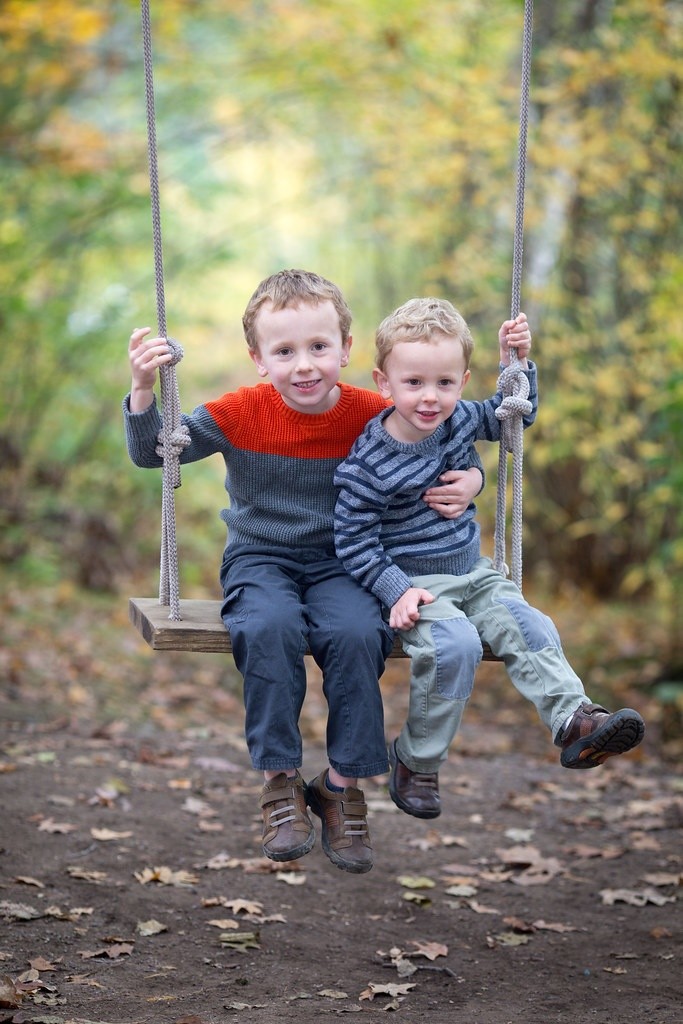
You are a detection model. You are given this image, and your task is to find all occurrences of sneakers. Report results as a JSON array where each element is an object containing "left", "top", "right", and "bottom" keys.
[
  {"left": 259, "top": 769, "right": 317, "bottom": 864},
  {"left": 560, "top": 703, "right": 646, "bottom": 770},
  {"left": 388, "top": 736, "right": 442, "bottom": 820},
  {"left": 308, "top": 767, "right": 374, "bottom": 874}
]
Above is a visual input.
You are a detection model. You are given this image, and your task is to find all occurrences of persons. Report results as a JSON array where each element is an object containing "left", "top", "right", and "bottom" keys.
[
  {"left": 335, "top": 297, "right": 645, "bottom": 820},
  {"left": 123, "top": 268, "right": 533, "bottom": 874}
]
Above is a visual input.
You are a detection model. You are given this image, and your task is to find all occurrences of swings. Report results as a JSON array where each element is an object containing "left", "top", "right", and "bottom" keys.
[{"left": 127, "top": 1, "right": 534, "bottom": 665}]
